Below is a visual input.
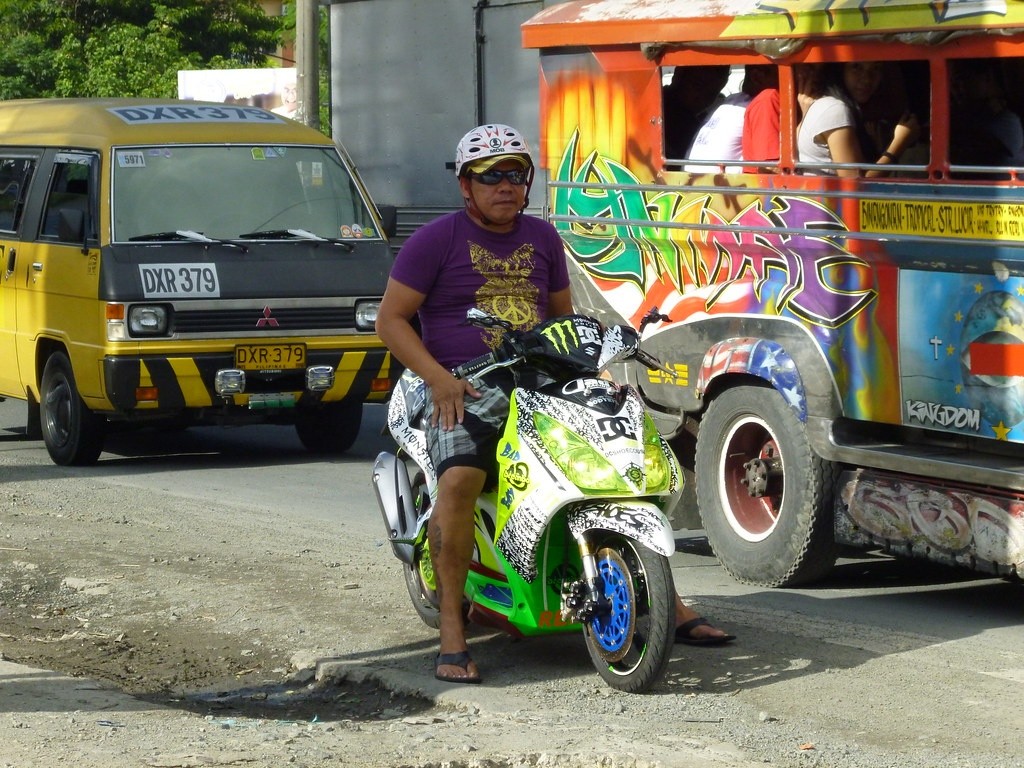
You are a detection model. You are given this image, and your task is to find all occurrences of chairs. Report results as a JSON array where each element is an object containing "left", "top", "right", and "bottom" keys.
[{"left": 41, "top": 192, "right": 97, "bottom": 240}]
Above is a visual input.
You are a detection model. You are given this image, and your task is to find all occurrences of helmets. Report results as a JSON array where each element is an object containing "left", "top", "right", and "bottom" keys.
[{"left": 446, "top": 124, "right": 535, "bottom": 178}]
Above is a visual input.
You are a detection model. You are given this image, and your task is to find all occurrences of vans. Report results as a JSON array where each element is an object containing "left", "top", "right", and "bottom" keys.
[
  {"left": 1, "top": 96, "right": 395, "bottom": 466},
  {"left": 519, "top": 0, "right": 1024, "bottom": 580}
]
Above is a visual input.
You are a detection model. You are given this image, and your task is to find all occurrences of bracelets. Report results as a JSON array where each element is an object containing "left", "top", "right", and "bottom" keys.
[{"left": 882, "top": 151, "right": 896, "bottom": 162}]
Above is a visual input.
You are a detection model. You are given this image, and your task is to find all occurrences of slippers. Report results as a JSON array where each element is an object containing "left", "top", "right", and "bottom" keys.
[{"left": 434, "top": 652, "right": 481, "bottom": 684}]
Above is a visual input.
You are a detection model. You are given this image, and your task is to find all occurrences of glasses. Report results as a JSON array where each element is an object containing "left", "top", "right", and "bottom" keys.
[{"left": 467, "top": 169, "right": 526, "bottom": 185}]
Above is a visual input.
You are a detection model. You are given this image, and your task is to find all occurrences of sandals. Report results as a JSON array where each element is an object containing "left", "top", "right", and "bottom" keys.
[{"left": 675, "top": 617, "right": 737, "bottom": 646}]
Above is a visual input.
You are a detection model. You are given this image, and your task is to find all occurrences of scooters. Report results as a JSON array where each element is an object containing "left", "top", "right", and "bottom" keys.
[{"left": 372, "top": 313, "right": 685, "bottom": 691}]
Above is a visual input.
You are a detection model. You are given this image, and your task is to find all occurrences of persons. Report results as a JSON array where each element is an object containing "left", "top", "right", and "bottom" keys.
[
  {"left": 374, "top": 124, "right": 738, "bottom": 683},
  {"left": 663, "top": 62, "right": 1024, "bottom": 178}
]
